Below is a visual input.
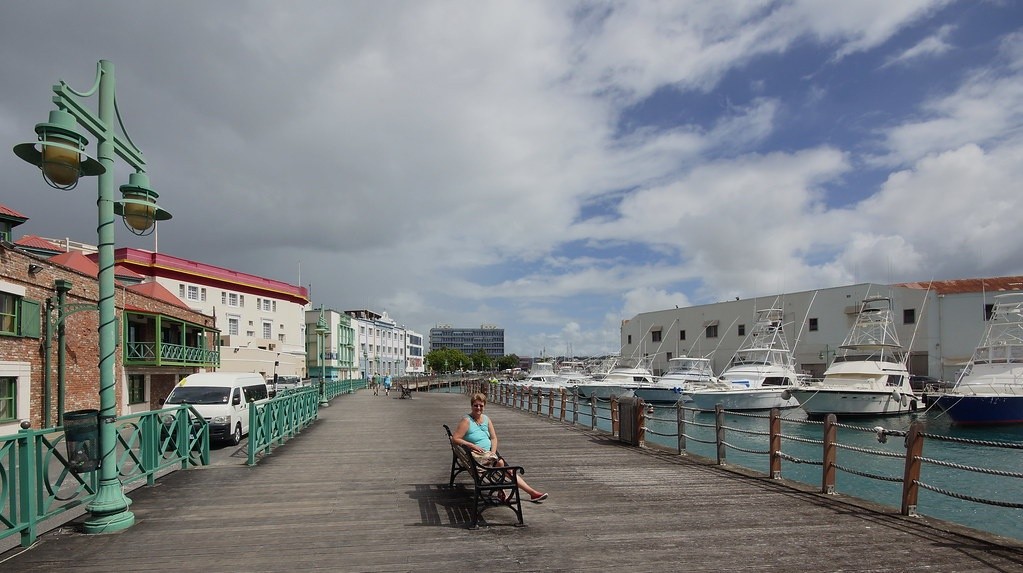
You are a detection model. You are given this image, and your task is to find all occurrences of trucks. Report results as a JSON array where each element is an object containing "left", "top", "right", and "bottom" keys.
[{"left": 275, "top": 374, "right": 312, "bottom": 399}]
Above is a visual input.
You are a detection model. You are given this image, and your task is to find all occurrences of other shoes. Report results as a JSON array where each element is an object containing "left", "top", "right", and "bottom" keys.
[
  {"left": 374, "top": 392, "right": 378, "bottom": 395},
  {"left": 386, "top": 392, "right": 389, "bottom": 397}
]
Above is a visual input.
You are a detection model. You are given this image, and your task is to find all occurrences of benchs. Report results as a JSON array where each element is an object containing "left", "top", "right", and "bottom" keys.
[
  {"left": 443, "top": 424, "right": 528, "bottom": 529},
  {"left": 400, "top": 384, "right": 412, "bottom": 400}
]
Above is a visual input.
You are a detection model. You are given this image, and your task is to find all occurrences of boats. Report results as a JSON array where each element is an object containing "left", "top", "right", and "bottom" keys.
[
  {"left": 505, "top": 363, "right": 595, "bottom": 396},
  {"left": 575, "top": 368, "right": 655, "bottom": 401},
  {"left": 678, "top": 307, "right": 813, "bottom": 412},
  {"left": 925, "top": 293, "right": 1023, "bottom": 426},
  {"left": 789, "top": 298, "right": 926, "bottom": 418},
  {"left": 623, "top": 357, "right": 713, "bottom": 404}
]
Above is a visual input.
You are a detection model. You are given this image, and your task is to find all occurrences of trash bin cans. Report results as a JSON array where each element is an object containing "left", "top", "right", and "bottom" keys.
[{"left": 63, "top": 409, "right": 101, "bottom": 474}]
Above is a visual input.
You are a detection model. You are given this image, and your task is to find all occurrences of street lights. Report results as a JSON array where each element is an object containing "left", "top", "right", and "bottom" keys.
[
  {"left": 363, "top": 349, "right": 369, "bottom": 388},
  {"left": 11, "top": 58, "right": 173, "bottom": 533},
  {"left": 314, "top": 303, "right": 333, "bottom": 409},
  {"left": 345, "top": 341, "right": 355, "bottom": 394}
]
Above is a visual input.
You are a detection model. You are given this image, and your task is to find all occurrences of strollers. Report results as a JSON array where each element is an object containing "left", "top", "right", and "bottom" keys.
[{"left": 399, "top": 384, "right": 412, "bottom": 399}]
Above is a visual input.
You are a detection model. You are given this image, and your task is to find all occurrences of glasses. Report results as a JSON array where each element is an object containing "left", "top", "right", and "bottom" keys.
[{"left": 472, "top": 404, "right": 484, "bottom": 407}]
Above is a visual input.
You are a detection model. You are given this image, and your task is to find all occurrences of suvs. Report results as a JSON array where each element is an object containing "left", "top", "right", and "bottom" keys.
[{"left": 264, "top": 378, "right": 279, "bottom": 397}]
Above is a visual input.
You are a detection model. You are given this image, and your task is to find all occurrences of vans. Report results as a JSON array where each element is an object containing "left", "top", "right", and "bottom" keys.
[{"left": 159, "top": 371, "right": 270, "bottom": 446}]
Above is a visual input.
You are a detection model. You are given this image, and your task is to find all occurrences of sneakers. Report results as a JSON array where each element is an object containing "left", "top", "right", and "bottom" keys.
[
  {"left": 497, "top": 490, "right": 509, "bottom": 502},
  {"left": 531, "top": 491, "right": 548, "bottom": 502}
]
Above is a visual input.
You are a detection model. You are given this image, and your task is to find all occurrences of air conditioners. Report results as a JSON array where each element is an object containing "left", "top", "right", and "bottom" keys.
[{"left": 270, "top": 344, "right": 275, "bottom": 348}]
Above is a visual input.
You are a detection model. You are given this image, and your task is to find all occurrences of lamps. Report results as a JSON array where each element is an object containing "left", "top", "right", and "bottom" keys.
[{"left": 27, "top": 265, "right": 43, "bottom": 273}]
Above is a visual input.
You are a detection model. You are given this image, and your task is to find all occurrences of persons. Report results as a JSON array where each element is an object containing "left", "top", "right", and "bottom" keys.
[
  {"left": 384, "top": 372, "right": 392, "bottom": 397},
  {"left": 367, "top": 371, "right": 381, "bottom": 396},
  {"left": 451, "top": 393, "right": 548, "bottom": 502}
]
